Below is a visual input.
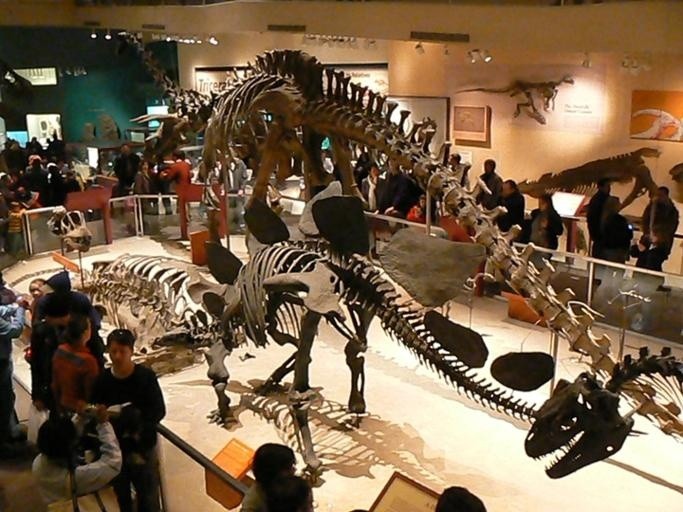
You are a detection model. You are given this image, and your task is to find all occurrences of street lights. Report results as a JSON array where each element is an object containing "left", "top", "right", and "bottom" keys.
[{"left": 47, "top": 485, "right": 121, "bottom": 512}]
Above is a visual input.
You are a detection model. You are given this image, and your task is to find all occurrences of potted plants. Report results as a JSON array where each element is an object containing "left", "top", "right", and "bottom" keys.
[
  {"left": 368, "top": 472, "right": 441, "bottom": 512},
  {"left": 384, "top": 94, "right": 450, "bottom": 141}
]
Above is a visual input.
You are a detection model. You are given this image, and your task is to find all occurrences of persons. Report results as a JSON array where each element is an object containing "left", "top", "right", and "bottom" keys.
[
  {"left": 243, "top": 179, "right": 286, "bottom": 260},
  {"left": 1, "top": 272, "right": 164, "bottom": 512},
  {"left": 437, "top": 487, "right": 486, "bottom": 511},
  {"left": 269, "top": 477, "right": 313, "bottom": 512},
  {"left": 319, "top": 145, "right": 678, "bottom": 271},
  {"left": 0, "top": 131, "right": 247, "bottom": 254},
  {"left": 240, "top": 444, "right": 296, "bottom": 512}
]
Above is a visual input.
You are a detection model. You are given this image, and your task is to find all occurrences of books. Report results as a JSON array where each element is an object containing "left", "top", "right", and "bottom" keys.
[{"left": 205, "top": 439, "right": 256, "bottom": 510}]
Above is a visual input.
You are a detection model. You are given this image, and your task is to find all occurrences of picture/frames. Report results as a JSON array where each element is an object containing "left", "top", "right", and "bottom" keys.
[{"left": 86, "top": 143, "right": 144, "bottom": 172}]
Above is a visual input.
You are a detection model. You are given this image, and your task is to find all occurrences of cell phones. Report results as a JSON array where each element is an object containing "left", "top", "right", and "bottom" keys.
[{"left": 85, "top": 405, "right": 96, "bottom": 415}]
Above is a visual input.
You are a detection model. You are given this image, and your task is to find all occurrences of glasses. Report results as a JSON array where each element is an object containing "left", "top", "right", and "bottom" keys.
[
  {"left": 468, "top": 52, "right": 476, "bottom": 63},
  {"left": 443, "top": 45, "right": 449, "bottom": 56},
  {"left": 304, "top": 33, "right": 376, "bottom": 44},
  {"left": 105, "top": 29, "right": 112, "bottom": 40},
  {"left": 472, "top": 49, "right": 492, "bottom": 62},
  {"left": 150, "top": 32, "right": 219, "bottom": 46},
  {"left": 415, "top": 42, "right": 424, "bottom": 55},
  {"left": 90, "top": 28, "right": 97, "bottom": 39}
]
